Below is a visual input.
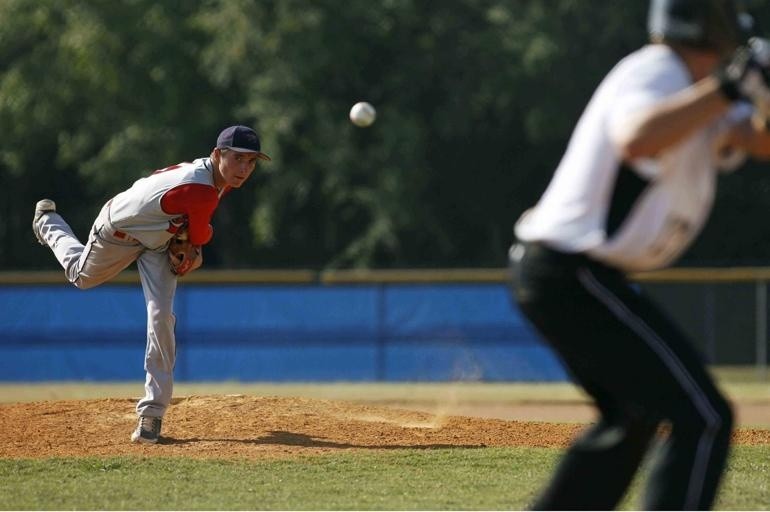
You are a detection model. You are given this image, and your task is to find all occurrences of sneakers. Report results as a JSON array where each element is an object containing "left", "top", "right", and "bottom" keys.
[
  {"left": 130, "top": 412, "right": 166, "bottom": 445},
  {"left": 32, "top": 198, "right": 57, "bottom": 248}
]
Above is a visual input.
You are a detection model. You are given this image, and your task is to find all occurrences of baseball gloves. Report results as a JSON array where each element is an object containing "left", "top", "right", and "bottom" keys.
[{"left": 171, "top": 232, "right": 203, "bottom": 276}]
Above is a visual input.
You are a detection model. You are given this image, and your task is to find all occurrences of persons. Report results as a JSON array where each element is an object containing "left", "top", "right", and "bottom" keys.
[
  {"left": 506, "top": 1, "right": 769, "bottom": 511},
  {"left": 33, "top": 123, "right": 270, "bottom": 445}
]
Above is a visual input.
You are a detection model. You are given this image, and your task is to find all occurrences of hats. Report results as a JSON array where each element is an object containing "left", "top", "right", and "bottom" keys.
[
  {"left": 646, "top": 1, "right": 736, "bottom": 53},
  {"left": 213, "top": 125, "right": 272, "bottom": 161}
]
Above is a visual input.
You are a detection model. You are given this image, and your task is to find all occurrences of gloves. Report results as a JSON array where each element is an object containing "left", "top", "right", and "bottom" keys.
[{"left": 717, "top": 36, "right": 770, "bottom": 103}]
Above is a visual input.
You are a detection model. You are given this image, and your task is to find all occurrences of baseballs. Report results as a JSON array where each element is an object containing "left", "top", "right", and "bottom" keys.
[{"left": 350, "top": 102, "right": 375, "bottom": 127}]
